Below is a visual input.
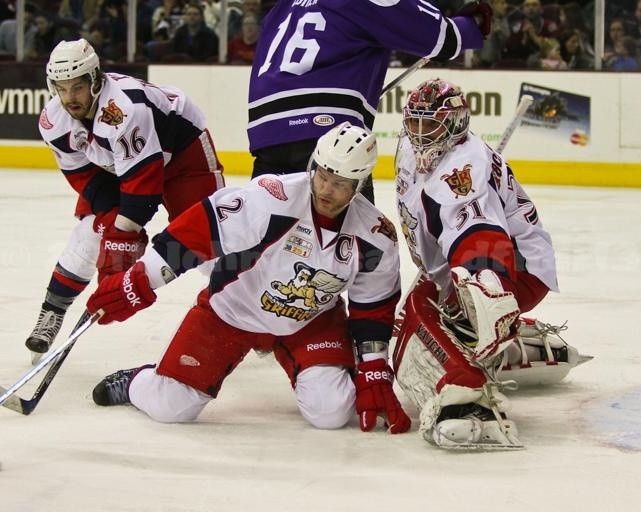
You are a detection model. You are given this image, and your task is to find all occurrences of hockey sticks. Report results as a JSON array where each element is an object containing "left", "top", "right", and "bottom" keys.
[{"left": 0, "top": 308, "right": 91, "bottom": 414}]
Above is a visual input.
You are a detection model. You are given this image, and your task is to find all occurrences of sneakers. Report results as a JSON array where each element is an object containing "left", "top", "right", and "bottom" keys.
[
  {"left": 432, "top": 413, "right": 518, "bottom": 447},
  {"left": 25, "top": 302, "right": 66, "bottom": 353},
  {"left": 559, "top": 345, "right": 579, "bottom": 368}
]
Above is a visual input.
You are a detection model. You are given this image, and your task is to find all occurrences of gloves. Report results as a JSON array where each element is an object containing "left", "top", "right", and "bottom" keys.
[
  {"left": 351, "top": 358, "right": 411, "bottom": 434},
  {"left": 86, "top": 207, "right": 157, "bottom": 324}
]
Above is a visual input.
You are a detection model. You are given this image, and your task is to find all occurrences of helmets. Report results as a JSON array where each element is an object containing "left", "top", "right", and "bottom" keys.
[
  {"left": 306, "top": 120, "right": 378, "bottom": 203},
  {"left": 402, "top": 78, "right": 471, "bottom": 175},
  {"left": 45, "top": 38, "right": 106, "bottom": 103}
]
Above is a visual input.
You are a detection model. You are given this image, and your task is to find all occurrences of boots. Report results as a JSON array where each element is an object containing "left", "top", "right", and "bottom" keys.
[{"left": 93, "top": 364, "right": 156, "bottom": 406}]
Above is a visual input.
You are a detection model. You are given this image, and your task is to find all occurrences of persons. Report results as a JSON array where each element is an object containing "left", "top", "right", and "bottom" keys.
[
  {"left": 390, "top": 76, "right": 580, "bottom": 452},
  {"left": 86, "top": 119, "right": 413, "bottom": 436},
  {"left": 25, "top": 39, "right": 226, "bottom": 356},
  {"left": 245, "top": 0, "right": 493, "bottom": 207},
  {"left": 1, "top": 1, "right": 641, "bottom": 71}
]
